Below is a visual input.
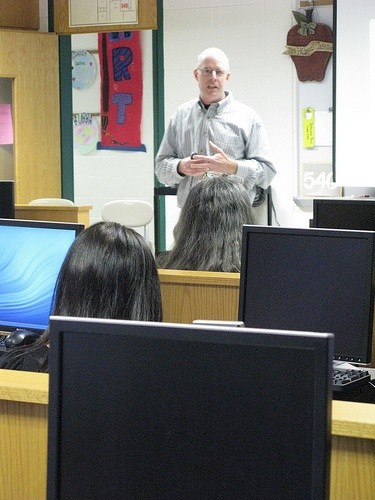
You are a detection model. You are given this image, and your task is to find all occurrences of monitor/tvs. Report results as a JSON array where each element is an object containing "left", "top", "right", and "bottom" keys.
[
  {"left": 47, "top": 314, "right": 334, "bottom": 498},
  {"left": 311, "top": 198, "right": 375, "bottom": 234},
  {"left": 236, "top": 223, "right": 375, "bottom": 367},
  {"left": 0, "top": 217, "right": 81, "bottom": 335}
]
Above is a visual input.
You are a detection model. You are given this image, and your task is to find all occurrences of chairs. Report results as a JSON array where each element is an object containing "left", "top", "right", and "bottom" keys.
[{"left": 102, "top": 200, "right": 153, "bottom": 242}]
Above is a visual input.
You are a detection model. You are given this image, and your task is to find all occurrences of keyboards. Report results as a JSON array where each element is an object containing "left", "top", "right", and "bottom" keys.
[{"left": 331, "top": 368, "right": 373, "bottom": 392}]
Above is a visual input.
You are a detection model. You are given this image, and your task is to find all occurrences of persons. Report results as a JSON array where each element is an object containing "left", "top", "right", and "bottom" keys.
[
  {"left": 154, "top": 47, "right": 277, "bottom": 205},
  {"left": 153, "top": 177, "right": 255, "bottom": 273},
  {"left": 0, "top": 221, "right": 163, "bottom": 374}
]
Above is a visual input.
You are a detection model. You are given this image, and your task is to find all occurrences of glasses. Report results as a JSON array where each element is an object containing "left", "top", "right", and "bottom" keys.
[
  {"left": 197, "top": 68, "right": 226, "bottom": 76},
  {"left": 202, "top": 171, "right": 244, "bottom": 184}
]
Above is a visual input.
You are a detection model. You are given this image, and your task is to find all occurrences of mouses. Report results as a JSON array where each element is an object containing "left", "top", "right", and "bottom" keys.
[{"left": 5, "top": 328, "right": 40, "bottom": 349}]
